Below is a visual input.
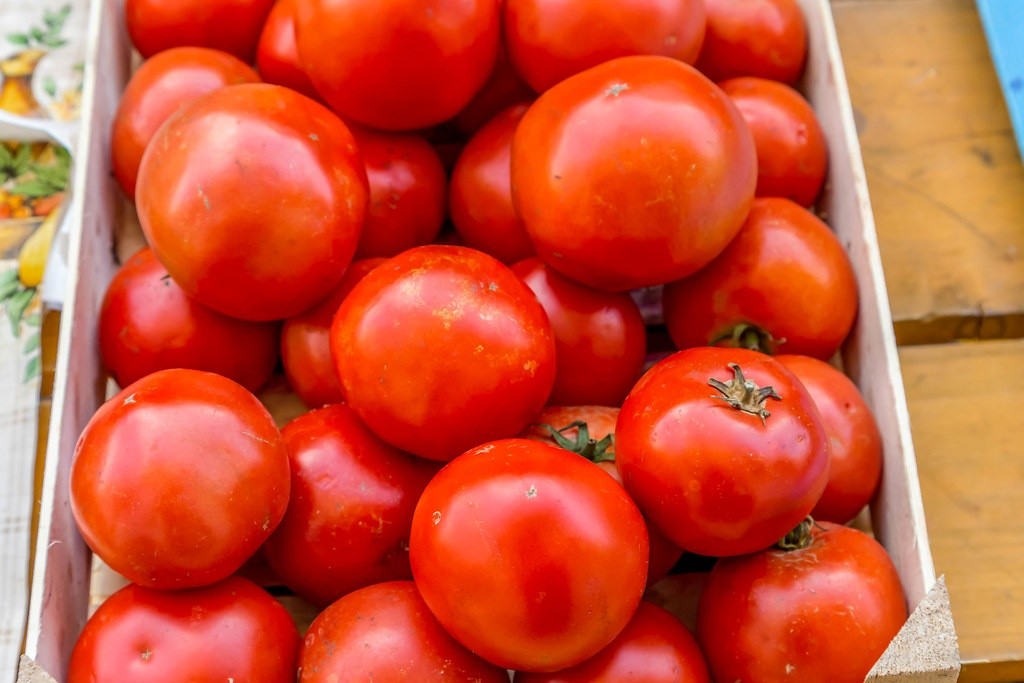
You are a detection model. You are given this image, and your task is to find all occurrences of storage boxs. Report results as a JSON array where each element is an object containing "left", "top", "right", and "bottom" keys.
[{"left": 22, "top": 2, "right": 961, "bottom": 683}]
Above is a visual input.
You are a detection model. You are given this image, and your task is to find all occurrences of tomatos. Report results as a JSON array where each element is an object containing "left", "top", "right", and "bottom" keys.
[{"left": 63, "top": 0, "right": 910, "bottom": 683}]
